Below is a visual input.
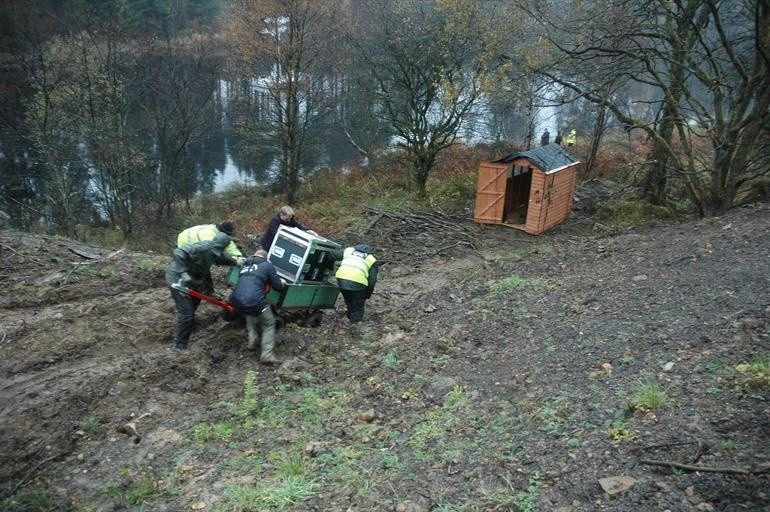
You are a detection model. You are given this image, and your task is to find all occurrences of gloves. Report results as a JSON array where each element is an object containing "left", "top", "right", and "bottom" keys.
[
  {"left": 181, "top": 272, "right": 192, "bottom": 283},
  {"left": 236, "top": 257, "right": 246, "bottom": 267}
]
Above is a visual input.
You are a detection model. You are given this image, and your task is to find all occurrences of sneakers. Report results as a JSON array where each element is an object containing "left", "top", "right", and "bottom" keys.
[{"left": 261, "top": 354, "right": 283, "bottom": 364}]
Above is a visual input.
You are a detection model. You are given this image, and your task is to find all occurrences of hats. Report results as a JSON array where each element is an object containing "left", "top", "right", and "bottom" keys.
[
  {"left": 250, "top": 249, "right": 268, "bottom": 258},
  {"left": 218, "top": 222, "right": 233, "bottom": 236}
]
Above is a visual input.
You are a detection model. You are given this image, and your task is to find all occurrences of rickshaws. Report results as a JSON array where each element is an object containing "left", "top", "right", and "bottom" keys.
[{"left": 171, "top": 258, "right": 342, "bottom": 335}]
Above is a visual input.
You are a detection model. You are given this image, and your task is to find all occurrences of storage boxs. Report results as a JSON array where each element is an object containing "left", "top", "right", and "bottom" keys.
[{"left": 266, "top": 223, "right": 342, "bottom": 285}]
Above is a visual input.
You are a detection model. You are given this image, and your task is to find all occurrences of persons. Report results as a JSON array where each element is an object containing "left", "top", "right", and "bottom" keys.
[
  {"left": 177, "top": 219, "right": 245, "bottom": 263},
  {"left": 165, "top": 232, "right": 245, "bottom": 349},
  {"left": 260, "top": 206, "right": 319, "bottom": 253},
  {"left": 567, "top": 128, "right": 577, "bottom": 146},
  {"left": 230, "top": 250, "right": 286, "bottom": 365},
  {"left": 555, "top": 130, "right": 562, "bottom": 145},
  {"left": 541, "top": 128, "right": 549, "bottom": 146},
  {"left": 326, "top": 244, "right": 378, "bottom": 323}
]
